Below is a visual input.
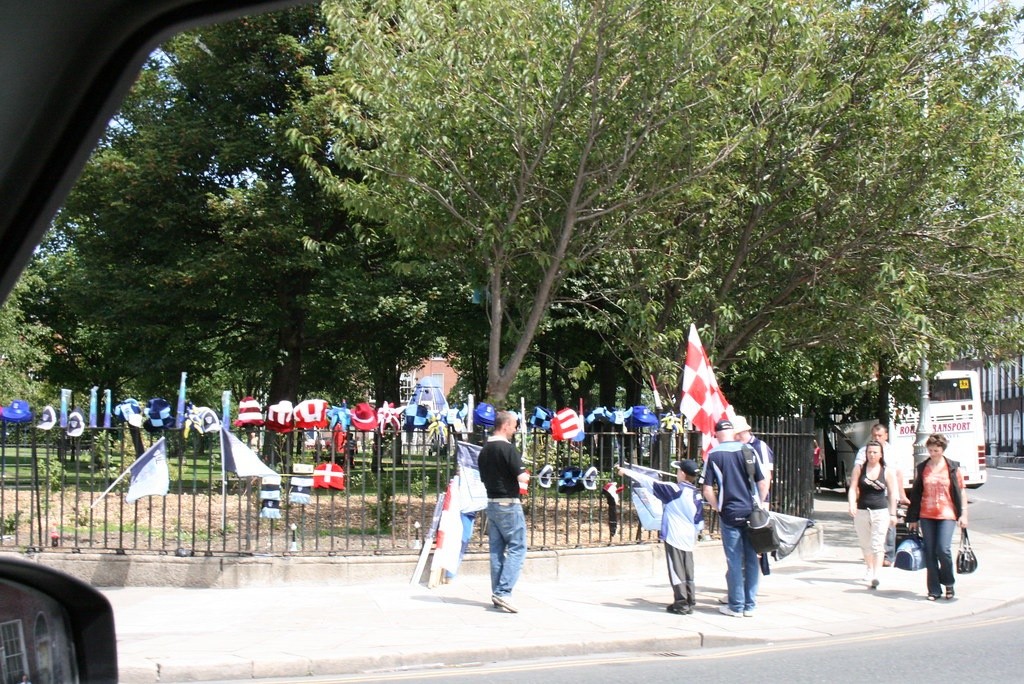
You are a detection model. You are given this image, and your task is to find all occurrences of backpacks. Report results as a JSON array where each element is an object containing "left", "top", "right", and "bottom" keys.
[{"left": 956, "top": 528, "right": 977, "bottom": 574}]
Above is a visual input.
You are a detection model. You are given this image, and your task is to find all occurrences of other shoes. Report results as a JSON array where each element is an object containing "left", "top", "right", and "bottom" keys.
[
  {"left": 719, "top": 605, "right": 753, "bottom": 617},
  {"left": 927, "top": 595, "right": 941, "bottom": 601},
  {"left": 872, "top": 578, "right": 879, "bottom": 588},
  {"left": 667, "top": 604, "right": 693, "bottom": 615},
  {"left": 492, "top": 594, "right": 518, "bottom": 612},
  {"left": 883, "top": 561, "right": 891, "bottom": 566},
  {"left": 719, "top": 595, "right": 729, "bottom": 603},
  {"left": 862, "top": 569, "right": 873, "bottom": 581},
  {"left": 946, "top": 586, "right": 954, "bottom": 599}
]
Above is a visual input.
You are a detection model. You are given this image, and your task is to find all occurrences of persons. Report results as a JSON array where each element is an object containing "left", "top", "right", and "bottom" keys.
[
  {"left": 699, "top": 416, "right": 774, "bottom": 618},
  {"left": 813, "top": 437, "right": 823, "bottom": 494},
  {"left": 478, "top": 410, "right": 527, "bottom": 613},
  {"left": 617, "top": 459, "right": 705, "bottom": 616},
  {"left": 848, "top": 424, "right": 968, "bottom": 602}
]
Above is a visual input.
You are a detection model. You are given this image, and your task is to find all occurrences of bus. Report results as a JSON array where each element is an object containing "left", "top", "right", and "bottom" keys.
[{"left": 802, "top": 368, "right": 988, "bottom": 492}]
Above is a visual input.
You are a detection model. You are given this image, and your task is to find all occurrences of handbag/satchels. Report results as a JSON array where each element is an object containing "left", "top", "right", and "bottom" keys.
[
  {"left": 746, "top": 508, "right": 780, "bottom": 554},
  {"left": 894, "top": 530, "right": 926, "bottom": 571}
]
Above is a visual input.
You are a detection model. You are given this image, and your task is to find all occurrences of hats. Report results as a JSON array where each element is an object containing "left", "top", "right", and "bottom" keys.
[
  {"left": 626, "top": 405, "right": 658, "bottom": 428},
  {"left": 1, "top": 398, "right": 330, "bottom": 437},
  {"left": 730, "top": 416, "right": 751, "bottom": 435},
  {"left": 474, "top": 402, "right": 496, "bottom": 427},
  {"left": 714, "top": 420, "right": 734, "bottom": 438},
  {"left": 351, "top": 403, "right": 378, "bottom": 430},
  {"left": 671, "top": 459, "right": 700, "bottom": 477}
]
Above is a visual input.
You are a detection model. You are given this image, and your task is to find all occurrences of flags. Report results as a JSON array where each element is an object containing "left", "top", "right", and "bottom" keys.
[
  {"left": 679, "top": 324, "right": 737, "bottom": 461},
  {"left": 125, "top": 440, "right": 169, "bottom": 503},
  {"left": 222, "top": 427, "right": 282, "bottom": 477}
]
